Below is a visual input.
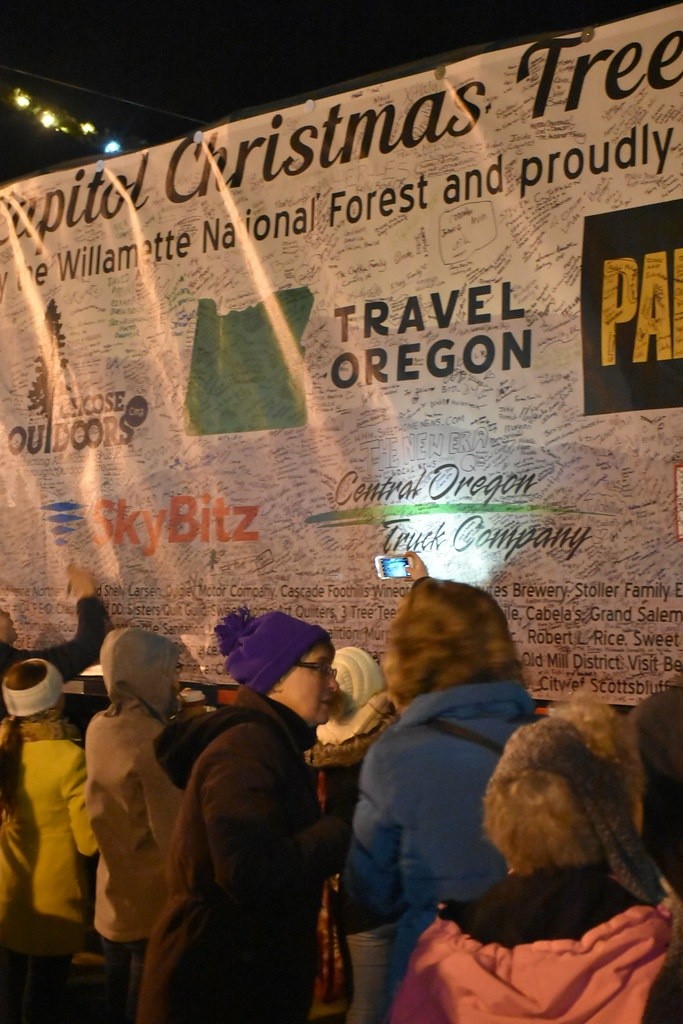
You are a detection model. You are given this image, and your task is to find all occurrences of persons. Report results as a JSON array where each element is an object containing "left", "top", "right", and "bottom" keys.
[{"left": 2, "top": 550, "right": 683, "bottom": 1024}]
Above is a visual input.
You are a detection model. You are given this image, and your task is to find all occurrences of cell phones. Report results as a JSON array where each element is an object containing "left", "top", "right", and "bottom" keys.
[{"left": 374, "top": 556, "right": 413, "bottom": 579}]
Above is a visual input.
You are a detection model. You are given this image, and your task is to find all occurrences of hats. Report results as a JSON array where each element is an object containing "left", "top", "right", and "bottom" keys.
[{"left": 328, "top": 646, "right": 387, "bottom": 723}]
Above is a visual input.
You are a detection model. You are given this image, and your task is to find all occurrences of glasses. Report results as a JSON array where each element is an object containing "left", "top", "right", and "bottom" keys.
[{"left": 295, "top": 660, "right": 339, "bottom": 682}]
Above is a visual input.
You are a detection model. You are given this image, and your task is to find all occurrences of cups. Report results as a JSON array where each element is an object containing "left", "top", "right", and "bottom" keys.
[{"left": 177, "top": 690, "right": 205, "bottom": 718}]
